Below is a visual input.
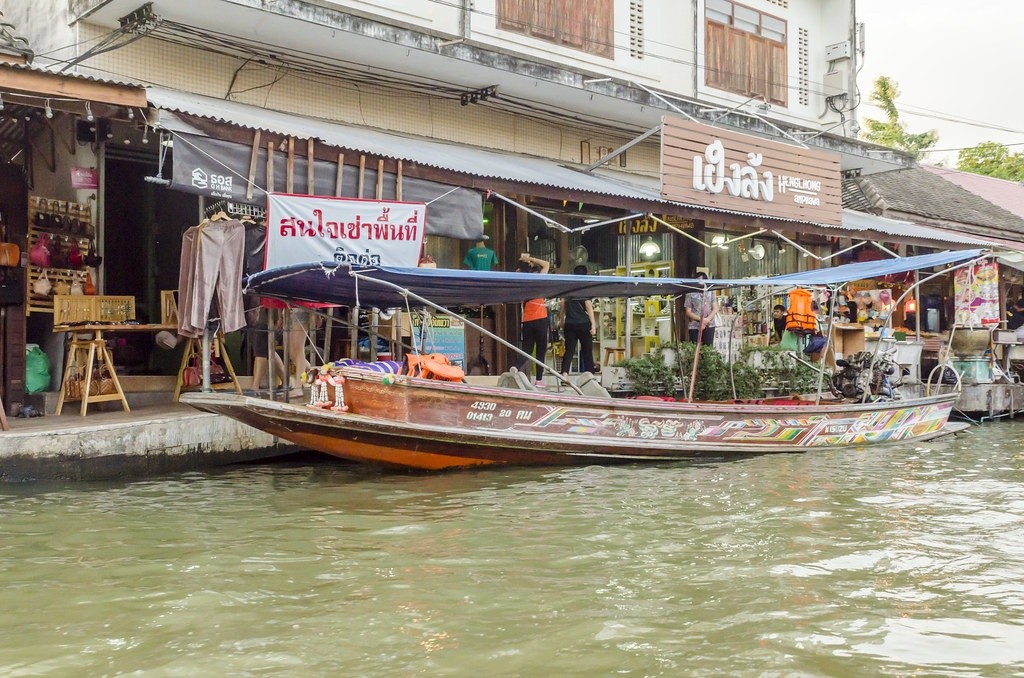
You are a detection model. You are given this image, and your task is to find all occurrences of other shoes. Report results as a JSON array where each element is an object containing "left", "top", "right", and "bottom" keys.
[
  {"left": 561, "top": 380, "right": 569, "bottom": 386},
  {"left": 243, "top": 389, "right": 261, "bottom": 399},
  {"left": 276, "top": 381, "right": 294, "bottom": 393},
  {"left": 288, "top": 388, "right": 303, "bottom": 397},
  {"left": 593, "top": 365, "right": 600, "bottom": 372},
  {"left": 536, "top": 382, "right": 546, "bottom": 388}
]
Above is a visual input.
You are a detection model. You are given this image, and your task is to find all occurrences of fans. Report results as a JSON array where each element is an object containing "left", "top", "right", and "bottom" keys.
[{"left": 567, "top": 244, "right": 589, "bottom": 271}]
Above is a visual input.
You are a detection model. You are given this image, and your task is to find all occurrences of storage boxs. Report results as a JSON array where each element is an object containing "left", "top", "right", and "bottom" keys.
[{"left": 811, "top": 322, "right": 866, "bottom": 375}]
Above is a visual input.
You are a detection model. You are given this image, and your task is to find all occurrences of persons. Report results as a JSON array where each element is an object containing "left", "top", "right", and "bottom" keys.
[
  {"left": 516, "top": 254, "right": 596, "bottom": 388},
  {"left": 465, "top": 241, "right": 500, "bottom": 273},
  {"left": 1005, "top": 298, "right": 1024, "bottom": 330},
  {"left": 248, "top": 294, "right": 311, "bottom": 399},
  {"left": 772, "top": 304, "right": 787, "bottom": 343},
  {"left": 684, "top": 272, "right": 718, "bottom": 348}
]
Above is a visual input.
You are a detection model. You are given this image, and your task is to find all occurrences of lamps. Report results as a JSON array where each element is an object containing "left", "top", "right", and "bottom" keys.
[
  {"left": 638, "top": 235, "right": 661, "bottom": 256},
  {"left": 711, "top": 232, "right": 731, "bottom": 250},
  {"left": 796, "top": 241, "right": 809, "bottom": 258},
  {"left": 748, "top": 237, "right": 756, "bottom": 252},
  {"left": 776, "top": 239, "right": 786, "bottom": 255}
]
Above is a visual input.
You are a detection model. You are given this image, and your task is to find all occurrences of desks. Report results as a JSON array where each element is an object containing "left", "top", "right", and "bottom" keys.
[{"left": 52, "top": 321, "right": 242, "bottom": 417}]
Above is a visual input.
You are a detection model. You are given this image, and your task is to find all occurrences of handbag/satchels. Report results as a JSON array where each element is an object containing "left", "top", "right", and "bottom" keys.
[
  {"left": 33, "top": 268, "right": 52, "bottom": 295},
  {"left": 0, "top": 267, "right": 23, "bottom": 305},
  {"left": 55, "top": 271, "right": 71, "bottom": 295},
  {"left": 65, "top": 364, "right": 117, "bottom": 396},
  {"left": 71, "top": 272, "right": 83, "bottom": 295},
  {"left": 84, "top": 273, "right": 96, "bottom": 295},
  {"left": 182, "top": 351, "right": 233, "bottom": 387},
  {"left": 30, "top": 232, "right": 102, "bottom": 270},
  {"left": 33, "top": 198, "right": 94, "bottom": 239},
  {"left": 25, "top": 347, "right": 51, "bottom": 393},
  {"left": 0, "top": 242, "right": 20, "bottom": 268}
]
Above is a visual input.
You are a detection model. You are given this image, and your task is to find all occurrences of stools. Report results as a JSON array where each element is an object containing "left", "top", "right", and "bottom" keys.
[{"left": 603, "top": 346, "right": 627, "bottom": 366}]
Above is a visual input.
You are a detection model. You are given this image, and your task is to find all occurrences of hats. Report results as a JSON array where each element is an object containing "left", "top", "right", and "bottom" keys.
[{"left": 854, "top": 290, "right": 891, "bottom": 322}]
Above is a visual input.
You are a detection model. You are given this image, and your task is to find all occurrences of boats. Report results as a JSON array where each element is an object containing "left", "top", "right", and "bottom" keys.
[{"left": 178, "top": 240, "right": 983, "bottom": 474}]
